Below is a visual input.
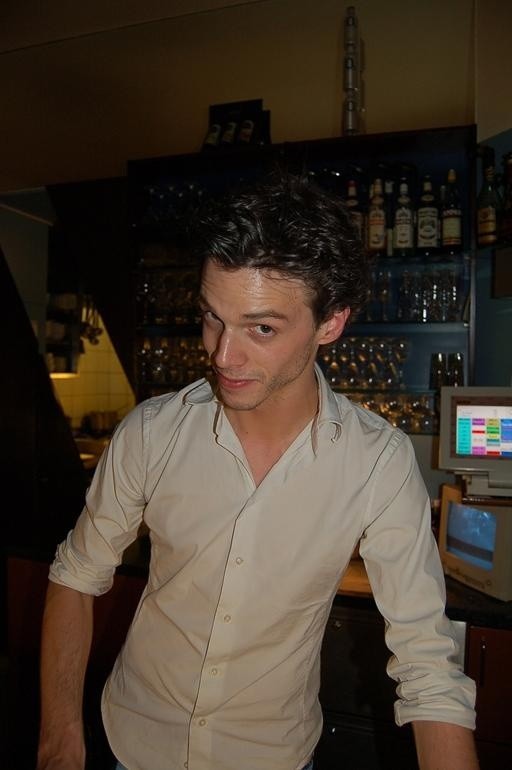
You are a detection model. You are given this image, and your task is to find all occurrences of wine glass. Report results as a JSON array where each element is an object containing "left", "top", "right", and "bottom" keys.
[
  {"left": 135, "top": 335, "right": 213, "bottom": 395},
  {"left": 363, "top": 254, "right": 465, "bottom": 321},
  {"left": 319, "top": 336, "right": 409, "bottom": 389}
]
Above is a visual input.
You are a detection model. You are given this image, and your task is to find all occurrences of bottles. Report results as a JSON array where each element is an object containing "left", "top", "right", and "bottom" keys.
[
  {"left": 352, "top": 394, "right": 436, "bottom": 433},
  {"left": 202, "top": 109, "right": 259, "bottom": 147},
  {"left": 429, "top": 352, "right": 464, "bottom": 392},
  {"left": 341, "top": 159, "right": 500, "bottom": 248}
]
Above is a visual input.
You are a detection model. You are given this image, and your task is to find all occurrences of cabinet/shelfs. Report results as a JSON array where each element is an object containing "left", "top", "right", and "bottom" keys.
[
  {"left": 466, "top": 624, "right": 512, "bottom": 770},
  {"left": 126, "top": 124, "right": 479, "bottom": 435},
  {"left": 313, "top": 593, "right": 418, "bottom": 769}
]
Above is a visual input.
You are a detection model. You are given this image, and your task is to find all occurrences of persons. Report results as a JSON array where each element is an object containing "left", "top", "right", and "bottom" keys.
[
  {"left": 33, "top": 179, "right": 481, "bottom": 769},
  {"left": 1, "top": 253, "right": 103, "bottom": 769}
]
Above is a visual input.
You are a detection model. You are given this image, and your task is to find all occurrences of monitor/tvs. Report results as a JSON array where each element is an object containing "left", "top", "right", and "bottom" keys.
[
  {"left": 437, "top": 386, "right": 512, "bottom": 497},
  {"left": 437, "top": 482, "right": 512, "bottom": 605}
]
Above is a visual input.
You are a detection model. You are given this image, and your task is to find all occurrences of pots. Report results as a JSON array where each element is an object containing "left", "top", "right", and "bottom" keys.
[{"left": 85, "top": 408, "right": 118, "bottom": 438}]
